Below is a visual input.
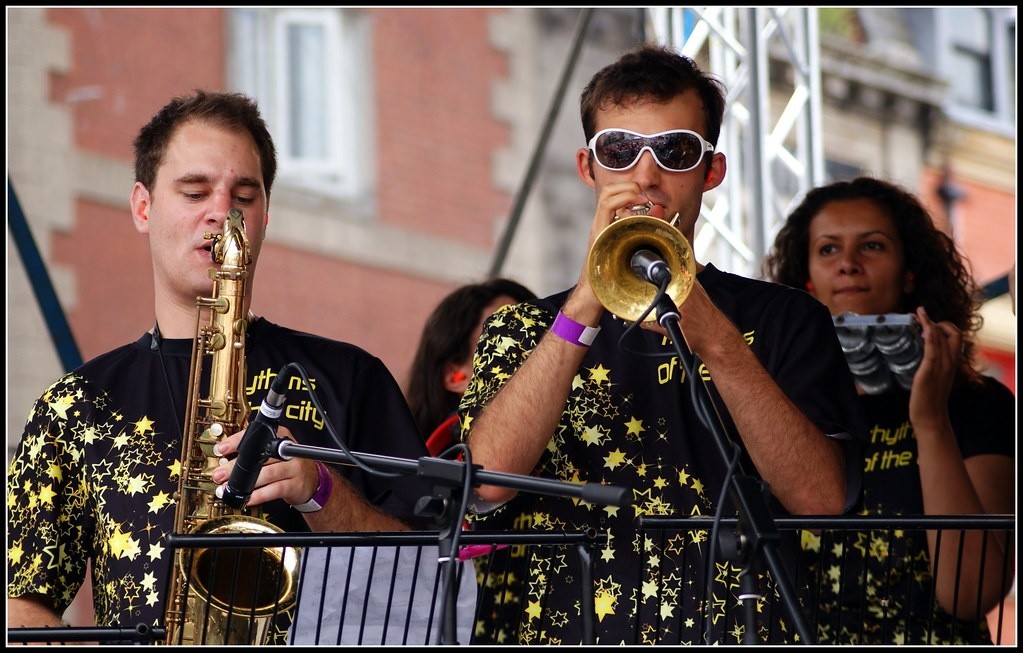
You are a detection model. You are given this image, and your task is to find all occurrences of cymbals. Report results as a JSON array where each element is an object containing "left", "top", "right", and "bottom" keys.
[{"left": 838, "top": 326, "right": 919, "bottom": 393}]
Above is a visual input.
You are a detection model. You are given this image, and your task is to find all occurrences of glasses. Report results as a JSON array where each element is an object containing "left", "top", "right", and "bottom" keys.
[{"left": 587, "top": 128, "right": 714, "bottom": 173}]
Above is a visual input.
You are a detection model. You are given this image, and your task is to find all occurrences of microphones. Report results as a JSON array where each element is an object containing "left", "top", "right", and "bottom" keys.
[
  {"left": 630, "top": 250, "right": 672, "bottom": 287},
  {"left": 221, "top": 365, "right": 294, "bottom": 512}
]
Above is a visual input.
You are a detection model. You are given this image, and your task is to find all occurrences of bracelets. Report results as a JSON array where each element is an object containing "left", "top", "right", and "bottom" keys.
[
  {"left": 551, "top": 310, "right": 601, "bottom": 347},
  {"left": 293, "top": 462, "right": 333, "bottom": 513}
]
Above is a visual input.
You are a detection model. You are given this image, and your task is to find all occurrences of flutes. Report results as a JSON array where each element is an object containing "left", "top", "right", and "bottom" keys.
[{"left": 587, "top": 204, "right": 696, "bottom": 323}]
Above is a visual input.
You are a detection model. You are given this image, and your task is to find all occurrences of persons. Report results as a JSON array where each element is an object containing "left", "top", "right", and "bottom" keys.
[
  {"left": 7, "top": 93, "right": 429, "bottom": 646},
  {"left": 762, "top": 178, "right": 1015, "bottom": 645},
  {"left": 409, "top": 278, "right": 540, "bottom": 444},
  {"left": 458, "top": 50, "right": 865, "bottom": 645}
]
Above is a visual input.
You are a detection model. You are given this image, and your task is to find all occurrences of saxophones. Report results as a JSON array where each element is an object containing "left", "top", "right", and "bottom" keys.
[{"left": 166, "top": 207, "right": 302, "bottom": 645}]
